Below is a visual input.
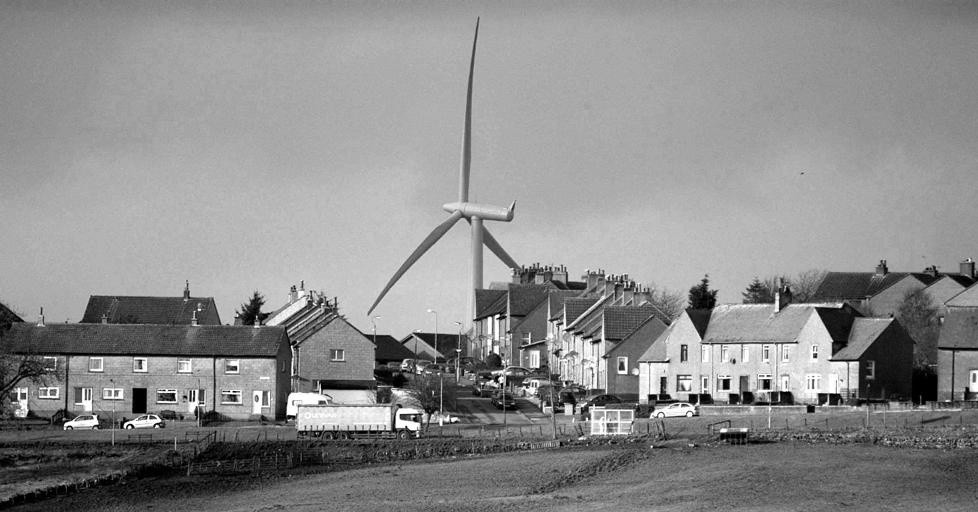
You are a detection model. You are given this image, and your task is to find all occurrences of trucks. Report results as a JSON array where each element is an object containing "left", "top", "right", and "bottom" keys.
[{"left": 299, "top": 404, "right": 423, "bottom": 441}]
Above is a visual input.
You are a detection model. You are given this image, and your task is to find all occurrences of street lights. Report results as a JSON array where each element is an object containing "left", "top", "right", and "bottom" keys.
[
  {"left": 427, "top": 308, "right": 437, "bottom": 365},
  {"left": 765, "top": 359, "right": 773, "bottom": 430},
  {"left": 455, "top": 321, "right": 462, "bottom": 383},
  {"left": 109, "top": 379, "right": 117, "bottom": 446},
  {"left": 374, "top": 315, "right": 381, "bottom": 342},
  {"left": 413, "top": 328, "right": 422, "bottom": 372},
  {"left": 195, "top": 378, "right": 201, "bottom": 422}
]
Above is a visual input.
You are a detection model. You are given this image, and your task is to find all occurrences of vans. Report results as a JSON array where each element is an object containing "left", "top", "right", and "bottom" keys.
[{"left": 286, "top": 392, "right": 333, "bottom": 421}]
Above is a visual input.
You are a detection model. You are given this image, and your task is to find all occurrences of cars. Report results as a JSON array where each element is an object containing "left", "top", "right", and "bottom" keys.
[
  {"left": 652, "top": 402, "right": 699, "bottom": 419},
  {"left": 63, "top": 414, "right": 102, "bottom": 430},
  {"left": 385, "top": 359, "right": 442, "bottom": 382},
  {"left": 472, "top": 364, "right": 621, "bottom": 416},
  {"left": 122, "top": 414, "right": 166, "bottom": 430}
]
